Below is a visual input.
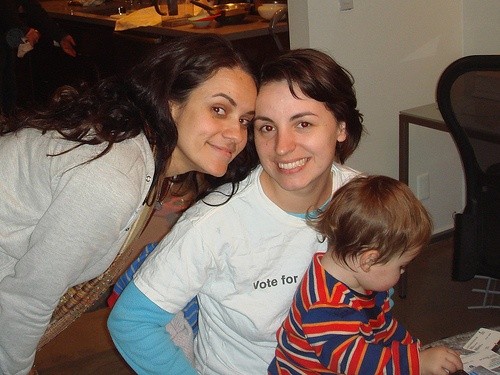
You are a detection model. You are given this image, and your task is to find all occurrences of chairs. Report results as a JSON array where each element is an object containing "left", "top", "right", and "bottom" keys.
[{"left": 436, "top": 56, "right": 500, "bottom": 282}]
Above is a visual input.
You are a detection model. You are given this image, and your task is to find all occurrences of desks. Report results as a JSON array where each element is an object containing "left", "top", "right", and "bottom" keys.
[
  {"left": 418, "top": 326, "right": 500, "bottom": 375},
  {"left": 399, "top": 102, "right": 450, "bottom": 186}
]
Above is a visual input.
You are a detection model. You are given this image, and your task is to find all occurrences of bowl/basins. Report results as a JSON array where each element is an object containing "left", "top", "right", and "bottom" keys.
[
  {"left": 208, "top": 4, "right": 254, "bottom": 23},
  {"left": 262, "top": 4, "right": 286, "bottom": 6},
  {"left": 258, "top": 6, "right": 287, "bottom": 21},
  {"left": 188, "top": 15, "right": 212, "bottom": 29}
]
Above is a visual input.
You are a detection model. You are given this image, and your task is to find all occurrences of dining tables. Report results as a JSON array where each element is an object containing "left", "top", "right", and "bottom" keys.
[{"left": 42, "top": 0, "right": 290, "bottom": 54}]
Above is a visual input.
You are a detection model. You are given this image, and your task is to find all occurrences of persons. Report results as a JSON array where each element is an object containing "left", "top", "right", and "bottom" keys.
[
  {"left": 0, "top": 0, "right": 96, "bottom": 106},
  {"left": 267, "top": 174, "right": 463, "bottom": 375},
  {"left": 106, "top": 48, "right": 370, "bottom": 375},
  {"left": 0, "top": 34, "right": 259, "bottom": 375}
]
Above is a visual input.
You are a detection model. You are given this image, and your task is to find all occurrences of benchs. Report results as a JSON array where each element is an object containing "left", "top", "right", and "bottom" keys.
[{"left": 34, "top": 306, "right": 137, "bottom": 375}]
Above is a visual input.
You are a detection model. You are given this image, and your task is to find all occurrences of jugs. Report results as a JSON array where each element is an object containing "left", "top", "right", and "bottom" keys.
[{"left": 154, "top": 0, "right": 195, "bottom": 27}]
